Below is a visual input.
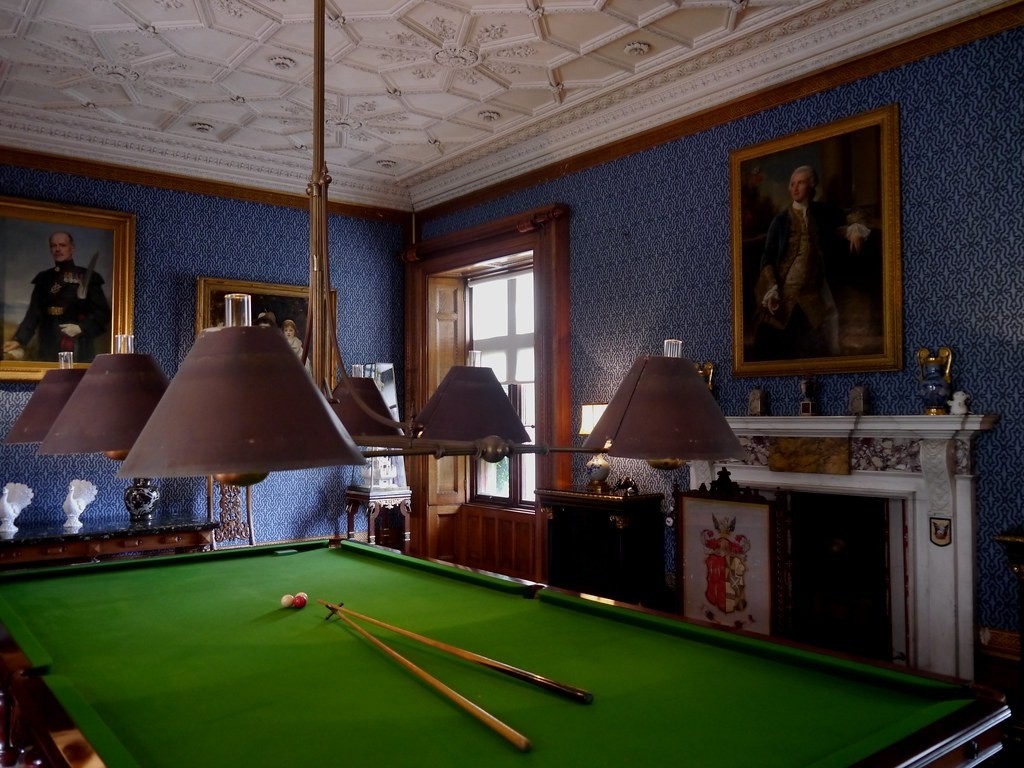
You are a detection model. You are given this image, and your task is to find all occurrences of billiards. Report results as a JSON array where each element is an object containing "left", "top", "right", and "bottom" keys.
[
  {"left": 295, "top": 591, "right": 309, "bottom": 602},
  {"left": 281, "top": 594, "right": 294, "bottom": 608},
  {"left": 292, "top": 596, "right": 308, "bottom": 609}
]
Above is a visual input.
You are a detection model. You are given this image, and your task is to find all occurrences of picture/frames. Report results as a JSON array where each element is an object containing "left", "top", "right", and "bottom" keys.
[
  {"left": 0, "top": 196, "right": 137, "bottom": 382},
  {"left": 731, "top": 103, "right": 903, "bottom": 370},
  {"left": 195, "top": 276, "right": 337, "bottom": 395}
]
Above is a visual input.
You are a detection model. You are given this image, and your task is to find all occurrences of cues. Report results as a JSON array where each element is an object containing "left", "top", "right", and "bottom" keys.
[
  {"left": 318, "top": 600, "right": 534, "bottom": 753},
  {"left": 315, "top": 596, "right": 596, "bottom": 706}
]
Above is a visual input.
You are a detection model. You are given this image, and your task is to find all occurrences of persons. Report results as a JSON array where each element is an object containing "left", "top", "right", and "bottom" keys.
[
  {"left": 211, "top": 302, "right": 312, "bottom": 382},
  {"left": 2, "top": 230, "right": 112, "bottom": 362}
]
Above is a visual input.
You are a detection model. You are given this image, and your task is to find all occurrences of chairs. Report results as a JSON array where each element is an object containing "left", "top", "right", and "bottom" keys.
[{"left": 671, "top": 467, "right": 792, "bottom": 640}]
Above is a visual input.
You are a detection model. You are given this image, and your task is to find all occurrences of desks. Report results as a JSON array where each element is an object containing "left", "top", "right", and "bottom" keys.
[
  {"left": 0, "top": 513, "right": 221, "bottom": 564},
  {"left": 347, "top": 491, "right": 412, "bottom": 552},
  {"left": 0, "top": 539, "right": 1011, "bottom": 768}
]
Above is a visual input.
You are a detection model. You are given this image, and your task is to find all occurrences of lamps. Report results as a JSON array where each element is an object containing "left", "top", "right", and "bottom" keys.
[
  {"left": 6, "top": 0, "right": 748, "bottom": 489},
  {"left": 578, "top": 402, "right": 612, "bottom": 494}
]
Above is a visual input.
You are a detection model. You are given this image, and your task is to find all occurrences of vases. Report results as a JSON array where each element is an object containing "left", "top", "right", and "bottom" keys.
[
  {"left": 917, "top": 347, "right": 952, "bottom": 414},
  {"left": 124, "top": 478, "right": 161, "bottom": 522}
]
